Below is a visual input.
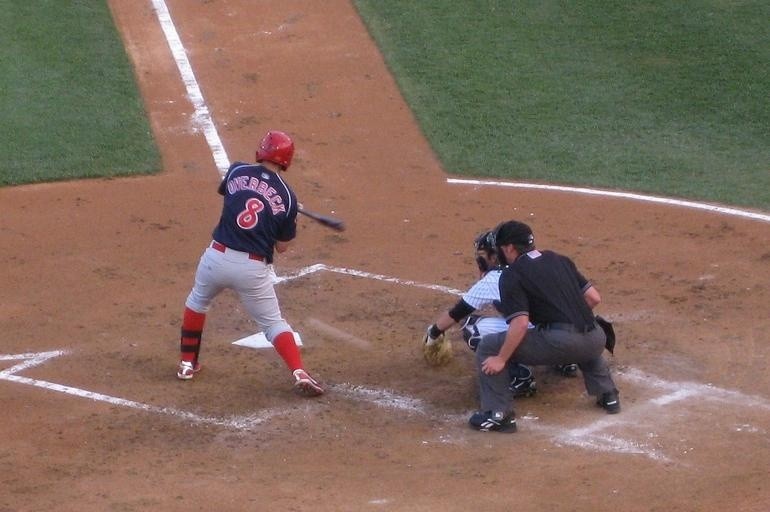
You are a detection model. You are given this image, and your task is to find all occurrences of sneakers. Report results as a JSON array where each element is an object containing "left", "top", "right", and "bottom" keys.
[
  {"left": 176, "top": 360, "right": 201, "bottom": 379},
  {"left": 470, "top": 410, "right": 516, "bottom": 432},
  {"left": 558, "top": 364, "right": 577, "bottom": 377},
  {"left": 293, "top": 369, "right": 324, "bottom": 395},
  {"left": 597, "top": 391, "right": 619, "bottom": 413},
  {"left": 509, "top": 375, "right": 537, "bottom": 398}
]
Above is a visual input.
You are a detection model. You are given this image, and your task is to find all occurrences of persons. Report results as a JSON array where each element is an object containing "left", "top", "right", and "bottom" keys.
[
  {"left": 175, "top": 126, "right": 325, "bottom": 398},
  {"left": 469, "top": 220, "right": 622, "bottom": 434},
  {"left": 429, "top": 230, "right": 582, "bottom": 399}
]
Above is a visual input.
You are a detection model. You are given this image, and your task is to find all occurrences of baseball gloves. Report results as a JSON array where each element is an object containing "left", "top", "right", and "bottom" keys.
[{"left": 422, "top": 323, "right": 454, "bottom": 365}]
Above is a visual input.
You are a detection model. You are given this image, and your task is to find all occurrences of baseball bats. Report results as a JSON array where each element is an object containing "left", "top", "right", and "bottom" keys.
[{"left": 297, "top": 206, "right": 348, "bottom": 231}]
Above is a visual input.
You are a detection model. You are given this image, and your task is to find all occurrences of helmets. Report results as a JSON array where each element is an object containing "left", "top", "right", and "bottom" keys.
[
  {"left": 473, "top": 222, "right": 534, "bottom": 272},
  {"left": 256, "top": 131, "right": 295, "bottom": 172}
]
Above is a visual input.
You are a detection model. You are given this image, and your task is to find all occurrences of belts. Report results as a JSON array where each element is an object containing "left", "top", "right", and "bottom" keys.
[{"left": 213, "top": 242, "right": 263, "bottom": 261}]
